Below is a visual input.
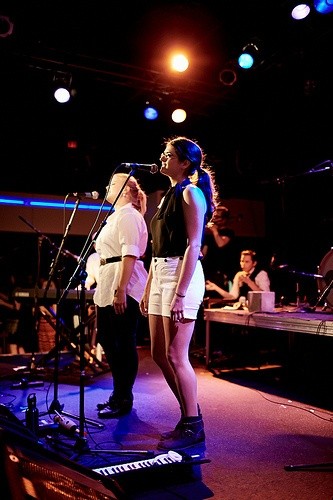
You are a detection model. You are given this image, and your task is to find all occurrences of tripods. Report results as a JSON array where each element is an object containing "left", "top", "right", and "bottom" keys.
[
  {"left": 47, "top": 171, "right": 155, "bottom": 463},
  {"left": 18, "top": 200, "right": 104, "bottom": 443}
]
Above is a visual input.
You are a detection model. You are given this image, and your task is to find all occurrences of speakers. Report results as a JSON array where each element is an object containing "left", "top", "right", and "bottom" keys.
[{"left": 247, "top": 291, "right": 276, "bottom": 312}]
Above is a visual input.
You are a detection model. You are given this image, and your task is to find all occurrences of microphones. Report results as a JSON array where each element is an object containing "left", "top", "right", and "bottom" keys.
[
  {"left": 68, "top": 190, "right": 99, "bottom": 200},
  {"left": 122, "top": 163, "right": 159, "bottom": 174},
  {"left": 62, "top": 249, "right": 85, "bottom": 266}
]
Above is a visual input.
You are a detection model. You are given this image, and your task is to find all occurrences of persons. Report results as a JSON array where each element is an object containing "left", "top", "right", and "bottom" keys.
[
  {"left": 0, "top": 232, "right": 98, "bottom": 354},
  {"left": 190, "top": 205, "right": 235, "bottom": 359},
  {"left": 140, "top": 136, "right": 216, "bottom": 452},
  {"left": 205, "top": 250, "right": 273, "bottom": 367},
  {"left": 94, "top": 174, "right": 150, "bottom": 419}
]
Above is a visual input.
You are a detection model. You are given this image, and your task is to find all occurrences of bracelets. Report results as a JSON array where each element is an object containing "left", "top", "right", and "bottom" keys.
[{"left": 175, "top": 292, "right": 186, "bottom": 297}]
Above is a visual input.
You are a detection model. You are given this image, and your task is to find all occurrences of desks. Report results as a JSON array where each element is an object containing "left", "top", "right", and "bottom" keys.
[{"left": 203, "top": 303, "right": 333, "bottom": 376}]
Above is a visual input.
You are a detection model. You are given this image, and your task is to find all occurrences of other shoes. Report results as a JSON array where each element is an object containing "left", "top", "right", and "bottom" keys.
[{"left": 96, "top": 400, "right": 133, "bottom": 418}]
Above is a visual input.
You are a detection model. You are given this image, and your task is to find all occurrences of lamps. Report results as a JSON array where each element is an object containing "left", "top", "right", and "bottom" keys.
[
  {"left": 43, "top": 64, "right": 75, "bottom": 108},
  {"left": 167, "top": 97, "right": 194, "bottom": 127},
  {"left": 288, "top": 0, "right": 333, "bottom": 24},
  {"left": 141, "top": 90, "right": 162, "bottom": 124},
  {"left": 237, "top": 38, "right": 264, "bottom": 72}
]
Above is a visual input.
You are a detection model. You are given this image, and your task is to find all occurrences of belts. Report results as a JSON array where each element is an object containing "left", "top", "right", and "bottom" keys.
[{"left": 100, "top": 255, "right": 141, "bottom": 265}]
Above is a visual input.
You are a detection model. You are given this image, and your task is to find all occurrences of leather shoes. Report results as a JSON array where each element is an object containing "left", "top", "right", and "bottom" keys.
[{"left": 156, "top": 416, "right": 206, "bottom": 451}]
